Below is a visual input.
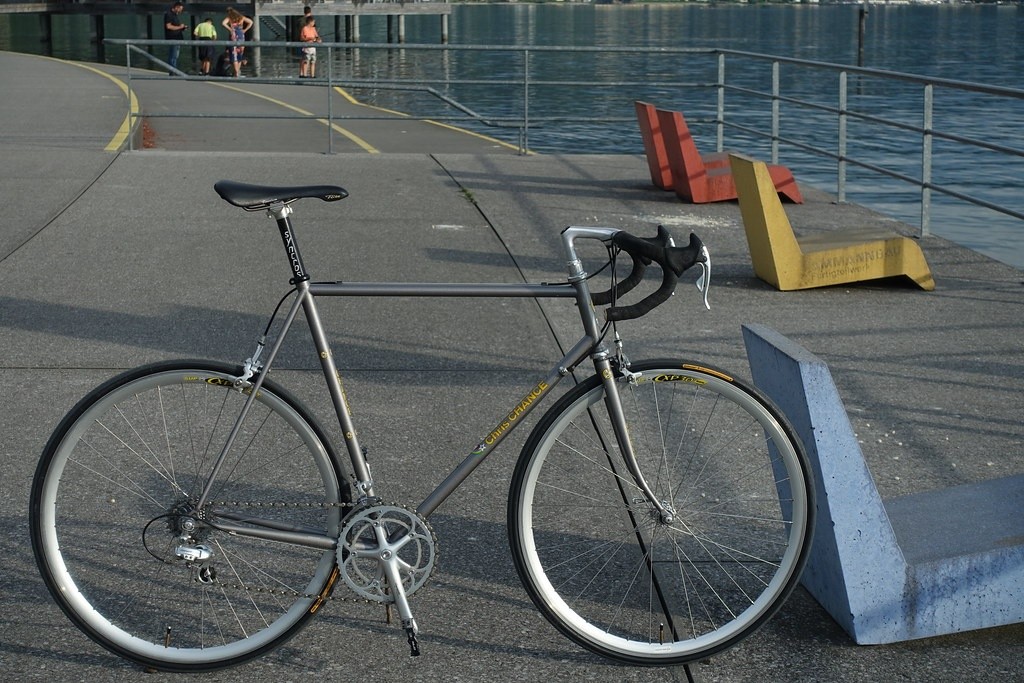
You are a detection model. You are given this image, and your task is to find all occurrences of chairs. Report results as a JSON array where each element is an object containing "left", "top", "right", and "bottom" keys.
[
  {"left": 729, "top": 151, "right": 935, "bottom": 292},
  {"left": 633, "top": 100, "right": 730, "bottom": 190},
  {"left": 742, "top": 323, "right": 1024, "bottom": 644},
  {"left": 656, "top": 107, "right": 805, "bottom": 206}
]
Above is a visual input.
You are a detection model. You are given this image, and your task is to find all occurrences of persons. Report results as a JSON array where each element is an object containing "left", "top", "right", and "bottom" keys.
[
  {"left": 194, "top": 18, "right": 217, "bottom": 76},
  {"left": 222, "top": 7, "right": 254, "bottom": 77},
  {"left": 164, "top": 1, "right": 190, "bottom": 77},
  {"left": 216, "top": 48, "right": 247, "bottom": 77},
  {"left": 297, "top": 7, "right": 317, "bottom": 77},
  {"left": 300, "top": 16, "right": 321, "bottom": 78}
]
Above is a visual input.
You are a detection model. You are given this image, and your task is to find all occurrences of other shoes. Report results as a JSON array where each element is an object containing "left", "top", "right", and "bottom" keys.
[{"left": 198, "top": 72, "right": 203, "bottom": 75}]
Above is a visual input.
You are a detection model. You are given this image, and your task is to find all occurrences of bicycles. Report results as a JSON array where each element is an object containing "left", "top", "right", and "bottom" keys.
[{"left": 28, "top": 180, "right": 818, "bottom": 672}]
